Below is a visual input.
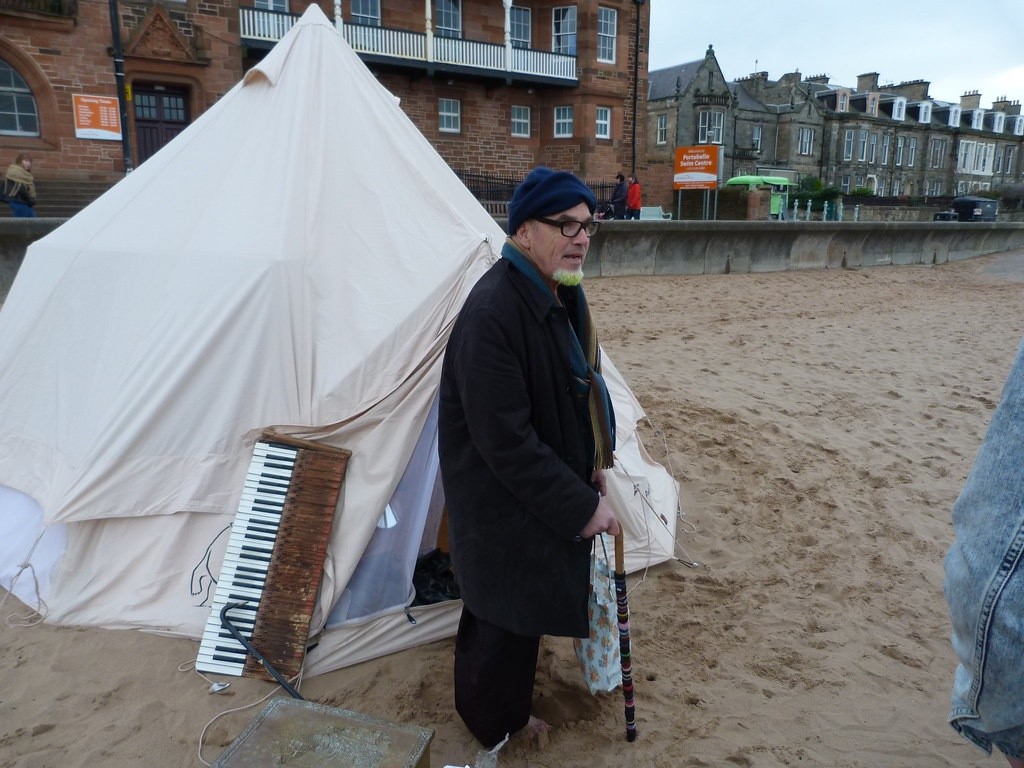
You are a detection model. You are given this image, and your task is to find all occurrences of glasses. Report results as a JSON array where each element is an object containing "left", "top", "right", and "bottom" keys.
[{"left": 534, "top": 218, "right": 601, "bottom": 238}]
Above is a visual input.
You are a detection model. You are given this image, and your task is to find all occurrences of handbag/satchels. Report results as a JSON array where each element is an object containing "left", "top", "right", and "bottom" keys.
[
  {"left": 343, "top": 547, "right": 460, "bottom": 622},
  {"left": 573, "top": 531, "right": 631, "bottom": 697}
]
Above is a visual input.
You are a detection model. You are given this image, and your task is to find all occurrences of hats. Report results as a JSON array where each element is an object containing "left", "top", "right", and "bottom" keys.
[
  {"left": 508, "top": 166, "right": 597, "bottom": 233},
  {"left": 628, "top": 173, "right": 637, "bottom": 181},
  {"left": 615, "top": 174, "right": 624, "bottom": 182}
]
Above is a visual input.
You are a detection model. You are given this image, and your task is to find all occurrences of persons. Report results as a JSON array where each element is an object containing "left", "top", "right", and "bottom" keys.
[
  {"left": 625, "top": 174, "right": 642, "bottom": 220},
  {"left": 939, "top": 340, "right": 1024, "bottom": 768},
  {"left": 4, "top": 153, "right": 39, "bottom": 217},
  {"left": 606, "top": 174, "right": 627, "bottom": 220},
  {"left": 437, "top": 169, "right": 619, "bottom": 751}
]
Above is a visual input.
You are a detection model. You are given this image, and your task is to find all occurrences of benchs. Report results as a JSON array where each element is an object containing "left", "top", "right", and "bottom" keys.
[{"left": 631, "top": 206, "right": 672, "bottom": 220}]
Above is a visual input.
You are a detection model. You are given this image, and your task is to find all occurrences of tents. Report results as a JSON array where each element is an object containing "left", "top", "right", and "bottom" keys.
[{"left": 0, "top": 3, "right": 680, "bottom": 683}]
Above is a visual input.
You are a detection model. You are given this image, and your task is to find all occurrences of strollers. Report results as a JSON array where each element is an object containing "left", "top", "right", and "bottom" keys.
[{"left": 593, "top": 200, "right": 615, "bottom": 220}]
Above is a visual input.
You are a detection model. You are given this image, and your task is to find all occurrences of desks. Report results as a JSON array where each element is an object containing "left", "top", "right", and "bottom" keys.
[{"left": 209, "top": 696, "right": 435, "bottom": 768}]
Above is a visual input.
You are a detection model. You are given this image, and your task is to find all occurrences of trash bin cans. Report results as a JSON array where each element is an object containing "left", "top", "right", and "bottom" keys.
[
  {"left": 952, "top": 196, "right": 999, "bottom": 222},
  {"left": 827, "top": 204, "right": 837, "bottom": 220},
  {"left": 933, "top": 212, "right": 959, "bottom": 221}
]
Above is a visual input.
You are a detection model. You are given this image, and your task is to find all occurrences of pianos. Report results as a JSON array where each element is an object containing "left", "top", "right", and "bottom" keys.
[{"left": 193, "top": 427, "right": 354, "bottom": 684}]
